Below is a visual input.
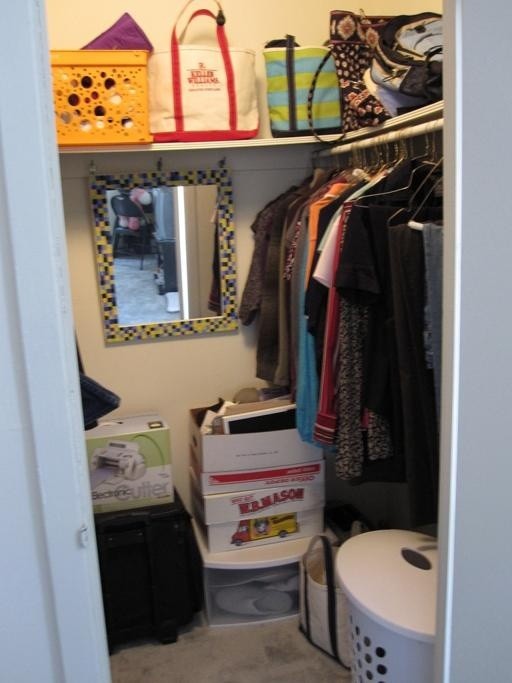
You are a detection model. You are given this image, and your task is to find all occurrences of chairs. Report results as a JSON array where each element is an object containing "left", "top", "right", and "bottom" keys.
[{"left": 110, "top": 195, "right": 160, "bottom": 271}]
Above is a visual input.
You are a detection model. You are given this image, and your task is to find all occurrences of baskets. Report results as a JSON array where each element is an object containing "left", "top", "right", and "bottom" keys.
[{"left": 49, "top": 49, "right": 153, "bottom": 147}]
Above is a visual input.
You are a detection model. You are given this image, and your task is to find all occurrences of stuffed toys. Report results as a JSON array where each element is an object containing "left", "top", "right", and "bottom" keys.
[{"left": 116, "top": 187, "right": 153, "bottom": 230}]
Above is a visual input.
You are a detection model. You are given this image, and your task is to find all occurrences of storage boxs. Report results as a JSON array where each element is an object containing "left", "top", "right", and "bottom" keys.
[
  {"left": 188, "top": 409, "right": 327, "bottom": 629},
  {"left": 85, "top": 412, "right": 175, "bottom": 515}
]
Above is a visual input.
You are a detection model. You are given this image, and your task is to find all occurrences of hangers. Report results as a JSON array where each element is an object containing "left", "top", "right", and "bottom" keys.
[{"left": 310, "top": 118, "right": 443, "bottom": 227}]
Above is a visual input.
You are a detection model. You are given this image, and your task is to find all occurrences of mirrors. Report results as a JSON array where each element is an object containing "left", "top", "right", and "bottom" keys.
[{"left": 88, "top": 168, "right": 240, "bottom": 347}]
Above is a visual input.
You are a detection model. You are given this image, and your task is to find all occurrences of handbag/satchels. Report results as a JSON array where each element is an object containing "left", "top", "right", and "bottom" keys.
[
  {"left": 149, "top": 46, "right": 259, "bottom": 142},
  {"left": 329, "top": 10, "right": 442, "bottom": 132},
  {"left": 93, "top": 486, "right": 204, "bottom": 655},
  {"left": 264, "top": 47, "right": 343, "bottom": 136},
  {"left": 299, "top": 533, "right": 354, "bottom": 670}
]
